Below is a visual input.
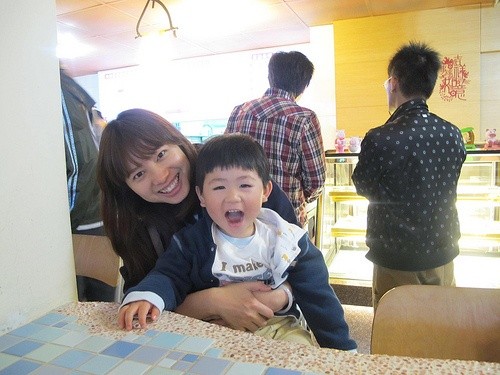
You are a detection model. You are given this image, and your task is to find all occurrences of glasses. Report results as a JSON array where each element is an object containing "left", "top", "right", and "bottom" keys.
[{"left": 383, "top": 76, "right": 394, "bottom": 90}]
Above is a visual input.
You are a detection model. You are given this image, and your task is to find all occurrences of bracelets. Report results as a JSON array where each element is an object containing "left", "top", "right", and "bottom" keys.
[{"left": 276, "top": 284, "right": 293, "bottom": 314}]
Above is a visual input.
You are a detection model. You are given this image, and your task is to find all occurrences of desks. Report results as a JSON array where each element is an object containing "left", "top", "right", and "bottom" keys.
[{"left": 0, "top": 302, "right": 500, "bottom": 375}]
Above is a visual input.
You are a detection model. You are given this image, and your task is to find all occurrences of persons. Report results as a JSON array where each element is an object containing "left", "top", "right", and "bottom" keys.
[
  {"left": 224, "top": 50, "right": 327, "bottom": 231},
  {"left": 95, "top": 108, "right": 357, "bottom": 353},
  {"left": 60, "top": 69, "right": 116, "bottom": 303},
  {"left": 352, "top": 41, "right": 467, "bottom": 312}
]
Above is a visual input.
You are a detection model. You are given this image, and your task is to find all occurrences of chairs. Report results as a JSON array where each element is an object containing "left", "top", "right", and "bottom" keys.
[
  {"left": 72, "top": 234, "right": 125, "bottom": 304},
  {"left": 370, "top": 285, "right": 500, "bottom": 363}
]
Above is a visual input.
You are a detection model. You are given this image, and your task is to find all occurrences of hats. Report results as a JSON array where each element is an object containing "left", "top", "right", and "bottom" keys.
[{"left": 58, "top": 48, "right": 72, "bottom": 71}]
